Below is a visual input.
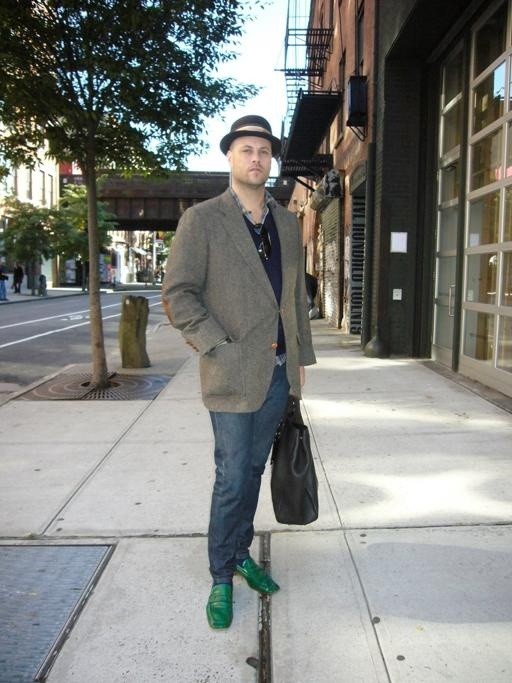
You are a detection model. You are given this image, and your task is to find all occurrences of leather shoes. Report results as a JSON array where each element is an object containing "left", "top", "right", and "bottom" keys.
[{"left": 206, "top": 558, "right": 279, "bottom": 628}]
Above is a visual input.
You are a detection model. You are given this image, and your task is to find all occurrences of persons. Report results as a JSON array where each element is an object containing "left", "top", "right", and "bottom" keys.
[
  {"left": 13, "top": 262, "right": 24, "bottom": 293},
  {"left": 162, "top": 115, "right": 320, "bottom": 631}
]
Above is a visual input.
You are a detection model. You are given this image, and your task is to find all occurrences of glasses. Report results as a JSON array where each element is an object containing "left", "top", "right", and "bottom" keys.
[{"left": 253, "top": 224, "right": 271, "bottom": 261}]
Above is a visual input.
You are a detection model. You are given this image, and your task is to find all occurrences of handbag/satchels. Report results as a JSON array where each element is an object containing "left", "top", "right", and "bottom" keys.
[{"left": 271, "top": 395, "right": 319, "bottom": 526}]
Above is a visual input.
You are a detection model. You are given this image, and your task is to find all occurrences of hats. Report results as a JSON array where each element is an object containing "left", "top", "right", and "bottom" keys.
[{"left": 220, "top": 115, "right": 281, "bottom": 156}]
